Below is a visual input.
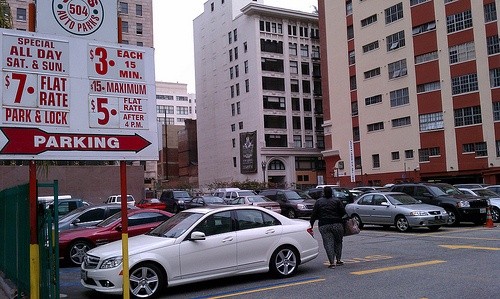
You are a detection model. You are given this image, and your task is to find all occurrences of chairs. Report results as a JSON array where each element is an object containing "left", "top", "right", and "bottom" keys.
[{"left": 204, "top": 217, "right": 231, "bottom": 237}]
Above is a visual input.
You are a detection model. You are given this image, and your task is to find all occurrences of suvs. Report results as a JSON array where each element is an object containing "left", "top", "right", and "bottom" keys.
[
  {"left": 390, "top": 182, "right": 488, "bottom": 227},
  {"left": 159, "top": 189, "right": 192, "bottom": 213},
  {"left": 258, "top": 185, "right": 354, "bottom": 218}
]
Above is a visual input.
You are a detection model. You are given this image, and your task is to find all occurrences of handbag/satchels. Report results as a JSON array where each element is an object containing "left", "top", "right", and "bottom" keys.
[{"left": 344, "top": 217, "right": 360, "bottom": 236}]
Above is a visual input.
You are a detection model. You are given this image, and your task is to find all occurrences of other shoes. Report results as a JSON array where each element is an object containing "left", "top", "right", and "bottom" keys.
[
  {"left": 336, "top": 261, "right": 344, "bottom": 266},
  {"left": 328, "top": 265, "right": 335, "bottom": 269}
]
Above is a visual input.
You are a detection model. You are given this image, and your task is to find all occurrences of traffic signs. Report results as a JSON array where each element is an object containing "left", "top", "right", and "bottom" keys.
[{"left": 0, "top": 27, "right": 159, "bottom": 160}]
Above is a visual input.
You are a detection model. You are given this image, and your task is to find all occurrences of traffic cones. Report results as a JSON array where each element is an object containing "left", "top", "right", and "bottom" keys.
[{"left": 482, "top": 208, "right": 497, "bottom": 228}]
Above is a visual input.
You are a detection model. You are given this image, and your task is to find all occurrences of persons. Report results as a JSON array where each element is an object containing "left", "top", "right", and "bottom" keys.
[{"left": 310, "top": 186, "right": 346, "bottom": 269}]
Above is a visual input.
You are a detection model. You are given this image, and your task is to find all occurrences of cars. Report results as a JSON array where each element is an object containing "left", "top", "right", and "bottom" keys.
[
  {"left": 449, "top": 183, "right": 500, "bottom": 223},
  {"left": 184, "top": 188, "right": 283, "bottom": 216},
  {"left": 80, "top": 205, "right": 322, "bottom": 299},
  {"left": 345, "top": 186, "right": 450, "bottom": 231},
  {"left": 37, "top": 195, "right": 178, "bottom": 266}
]
